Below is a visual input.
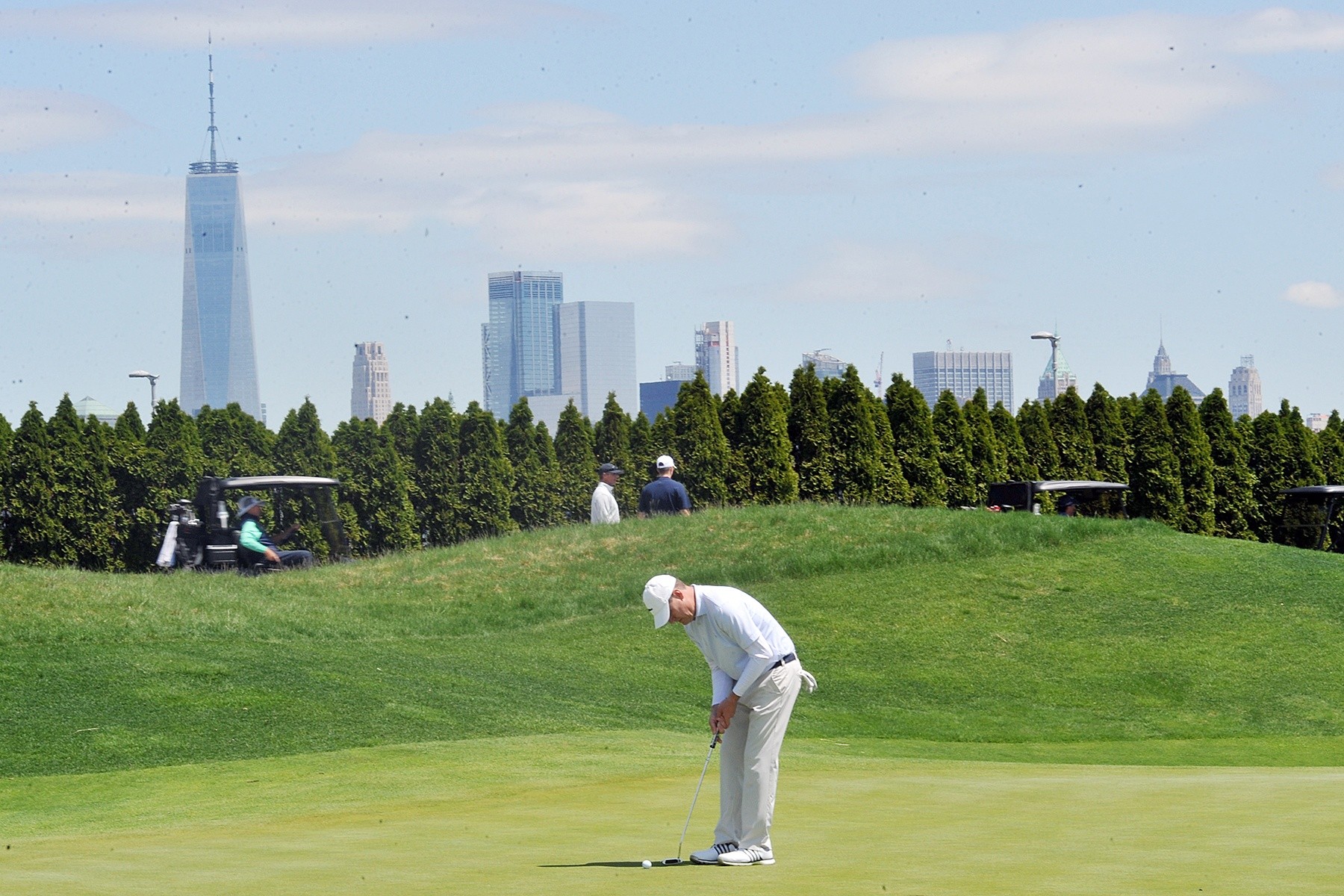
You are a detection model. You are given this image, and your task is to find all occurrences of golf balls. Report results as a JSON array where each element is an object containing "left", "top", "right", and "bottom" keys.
[{"left": 642, "top": 860, "right": 652, "bottom": 869}]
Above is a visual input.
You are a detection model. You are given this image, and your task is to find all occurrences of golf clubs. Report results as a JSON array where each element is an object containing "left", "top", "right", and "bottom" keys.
[
  {"left": 168, "top": 503, "right": 200, "bottom": 526},
  {"left": 661, "top": 720, "right": 720, "bottom": 865}
]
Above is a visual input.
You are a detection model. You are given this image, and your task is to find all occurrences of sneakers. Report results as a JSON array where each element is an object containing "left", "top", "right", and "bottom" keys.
[
  {"left": 718, "top": 848, "right": 776, "bottom": 866},
  {"left": 690, "top": 843, "right": 739, "bottom": 864}
]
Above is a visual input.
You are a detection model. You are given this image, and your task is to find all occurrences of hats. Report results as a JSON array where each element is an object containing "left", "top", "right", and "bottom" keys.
[
  {"left": 1057, "top": 494, "right": 1081, "bottom": 507},
  {"left": 235, "top": 495, "right": 268, "bottom": 520},
  {"left": 643, "top": 574, "right": 676, "bottom": 629},
  {"left": 599, "top": 463, "right": 623, "bottom": 475},
  {"left": 657, "top": 455, "right": 677, "bottom": 469}
]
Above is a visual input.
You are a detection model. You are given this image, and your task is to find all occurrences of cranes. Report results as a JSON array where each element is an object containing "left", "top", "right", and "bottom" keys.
[{"left": 874, "top": 351, "right": 883, "bottom": 399}]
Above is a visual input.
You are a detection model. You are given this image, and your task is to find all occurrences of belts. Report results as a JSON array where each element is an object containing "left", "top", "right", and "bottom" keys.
[{"left": 769, "top": 653, "right": 796, "bottom": 670}]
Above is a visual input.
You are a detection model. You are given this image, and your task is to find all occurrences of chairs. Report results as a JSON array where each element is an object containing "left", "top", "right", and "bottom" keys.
[{"left": 232, "top": 529, "right": 277, "bottom": 574}]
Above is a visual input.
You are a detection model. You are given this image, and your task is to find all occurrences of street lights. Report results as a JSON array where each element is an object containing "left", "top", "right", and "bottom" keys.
[
  {"left": 129, "top": 371, "right": 160, "bottom": 419},
  {"left": 1031, "top": 332, "right": 1058, "bottom": 396}
]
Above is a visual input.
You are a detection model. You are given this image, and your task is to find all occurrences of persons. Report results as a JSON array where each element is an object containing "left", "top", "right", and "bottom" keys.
[
  {"left": 637, "top": 455, "right": 692, "bottom": 521},
  {"left": 642, "top": 574, "right": 802, "bottom": 866},
  {"left": 235, "top": 496, "right": 314, "bottom": 570},
  {"left": 590, "top": 464, "right": 624, "bottom": 525},
  {"left": 1056, "top": 495, "right": 1078, "bottom": 516}
]
